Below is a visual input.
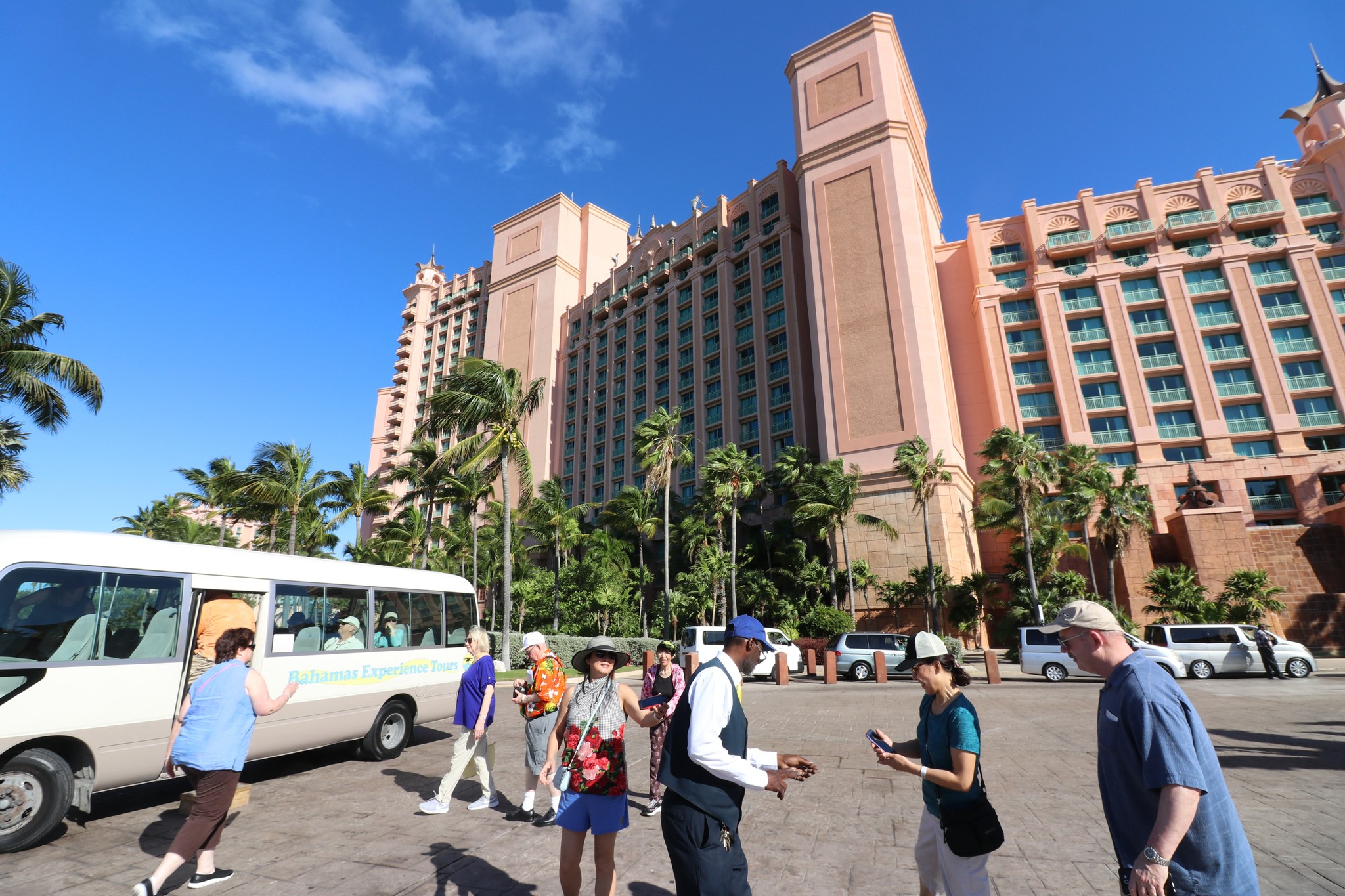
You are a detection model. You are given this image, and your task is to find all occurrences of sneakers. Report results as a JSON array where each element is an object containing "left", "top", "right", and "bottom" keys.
[
  {"left": 132, "top": 878, "right": 158, "bottom": 896},
  {"left": 643, "top": 797, "right": 662, "bottom": 816},
  {"left": 187, "top": 867, "right": 234, "bottom": 889},
  {"left": 468, "top": 793, "right": 499, "bottom": 810},
  {"left": 419, "top": 797, "right": 449, "bottom": 813}
]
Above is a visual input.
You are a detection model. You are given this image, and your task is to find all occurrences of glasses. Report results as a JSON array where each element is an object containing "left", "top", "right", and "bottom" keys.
[
  {"left": 526, "top": 644, "right": 536, "bottom": 655},
  {"left": 246, "top": 644, "right": 256, "bottom": 651},
  {"left": 657, "top": 652, "right": 671, "bottom": 656},
  {"left": 754, "top": 640, "right": 766, "bottom": 664},
  {"left": 592, "top": 650, "right": 618, "bottom": 660},
  {"left": 464, "top": 638, "right": 474, "bottom": 643},
  {"left": 910, "top": 662, "right": 927, "bottom": 675},
  {"left": 385, "top": 619, "right": 398, "bottom": 622},
  {"left": 287, "top": 619, "right": 303, "bottom": 625},
  {"left": 1057, "top": 632, "right": 1091, "bottom": 650},
  {"left": 341, "top": 621, "right": 354, "bottom": 627}
]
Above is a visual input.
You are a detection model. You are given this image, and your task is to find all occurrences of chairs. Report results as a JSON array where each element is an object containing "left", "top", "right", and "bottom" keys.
[
  {"left": 128, "top": 607, "right": 178, "bottom": 659},
  {"left": 0, "top": 618, "right": 34, "bottom": 658},
  {"left": 46, "top": 613, "right": 105, "bottom": 660},
  {"left": 354, "top": 626, "right": 367, "bottom": 648},
  {"left": 448, "top": 627, "right": 469, "bottom": 645},
  {"left": 292, "top": 626, "right": 323, "bottom": 651},
  {"left": 104, "top": 627, "right": 140, "bottom": 658},
  {"left": 393, "top": 624, "right": 411, "bottom": 647},
  {"left": 419, "top": 625, "right": 450, "bottom": 646}
]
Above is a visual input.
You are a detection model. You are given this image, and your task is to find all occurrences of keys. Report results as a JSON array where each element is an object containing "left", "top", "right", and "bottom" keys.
[{"left": 718, "top": 822, "right": 735, "bottom": 852}]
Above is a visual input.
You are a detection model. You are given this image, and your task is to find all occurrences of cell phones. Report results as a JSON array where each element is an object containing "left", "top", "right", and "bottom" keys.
[
  {"left": 866, "top": 728, "right": 897, "bottom": 756},
  {"left": 638, "top": 693, "right": 668, "bottom": 710},
  {"left": 1117, "top": 869, "right": 1175, "bottom": 896}
]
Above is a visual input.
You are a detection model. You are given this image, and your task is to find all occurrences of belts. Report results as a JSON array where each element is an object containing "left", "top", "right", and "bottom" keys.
[{"left": 525, "top": 705, "right": 560, "bottom": 722}]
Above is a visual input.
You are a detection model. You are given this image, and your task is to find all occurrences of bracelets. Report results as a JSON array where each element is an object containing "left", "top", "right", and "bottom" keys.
[
  {"left": 390, "top": 630, "right": 395, "bottom": 633},
  {"left": 654, "top": 713, "right": 667, "bottom": 722},
  {"left": 521, "top": 694, "right": 526, "bottom": 704},
  {"left": 920, "top": 766, "right": 928, "bottom": 779}
]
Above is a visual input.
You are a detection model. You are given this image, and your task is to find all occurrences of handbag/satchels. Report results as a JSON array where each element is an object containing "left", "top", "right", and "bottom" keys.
[
  {"left": 552, "top": 766, "right": 571, "bottom": 791},
  {"left": 937, "top": 792, "right": 1004, "bottom": 857},
  {"left": 462, "top": 742, "right": 496, "bottom": 779}
]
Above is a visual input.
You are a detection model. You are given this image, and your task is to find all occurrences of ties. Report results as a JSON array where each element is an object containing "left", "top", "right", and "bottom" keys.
[{"left": 736, "top": 681, "right": 743, "bottom": 708}]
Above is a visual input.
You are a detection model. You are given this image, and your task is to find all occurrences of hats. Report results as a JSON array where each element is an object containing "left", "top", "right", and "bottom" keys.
[
  {"left": 894, "top": 631, "right": 949, "bottom": 672},
  {"left": 517, "top": 631, "right": 545, "bottom": 653},
  {"left": 572, "top": 635, "right": 631, "bottom": 673},
  {"left": 1038, "top": 600, "right": 1125, "bottom": 635},
  {"left": 657, "top": 640, "right": 676, "bottom": 654},
  {"left": 724, "top": 614, "right": 777, "bottom": 651},
  {"left": 384, "top": 612, "right": 398, "bottom": 619},
  {"left": 337, "top": 616, "right": 360, "bottom": 630}
]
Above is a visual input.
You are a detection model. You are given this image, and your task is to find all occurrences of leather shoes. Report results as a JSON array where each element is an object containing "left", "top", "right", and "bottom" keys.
[
  {"left": 534, "top": 807, "right": 558, "bottom": 827},
  {"left": 1268, "top": 677, "right": 1277, "bottom": 680},
  {"left": 1280, "top": 677, "right": 1291, "bottom": 680},
  {"left": 506, "top": 804, "right": 535, "bottom": 822}
]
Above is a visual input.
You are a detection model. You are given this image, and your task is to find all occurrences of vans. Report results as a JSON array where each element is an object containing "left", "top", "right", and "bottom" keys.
[
  {"left": 1017, "top": 626, "right": 1189, "bottom": 689},
  {"left": 823, "top": 631, "right": 926, "bottom": 681},
  {"left": 1141, "top": 623, "right": 1318, "bottom": 678},
  {"left": 679, "top": 626, "right": 799, "bottom": 681}
]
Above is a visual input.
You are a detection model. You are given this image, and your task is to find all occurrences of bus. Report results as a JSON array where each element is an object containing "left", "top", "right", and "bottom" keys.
[{"left": 0, "top": 529, "right": 488, "bottom": 846}]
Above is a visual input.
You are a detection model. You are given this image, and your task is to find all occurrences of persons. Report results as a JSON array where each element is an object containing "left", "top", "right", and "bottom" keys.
[
  {"left": 1253, "top": 624, "right": 1292, "bottom": 679},
  {"left": 286, "top": 611, "right": 307, "bottom": 637},
  {"left": 418, "top": 623, "right": 501, "bottom": 814},
  {"left": 503, "top": 630, "right": 569, "bottom": 824},
  {"left": 6, "top": 570, "right": 97, "bottom": 659},
  {"left": 132, "top": 629, "right": 298, "bottom": 895},
  {"left": 654, "top": 613, "right": 820, "bottom": 896},
  {"left": 187, "top": 589, "right": 256, "bottom": 689},
  {"left": 867, "top": 630, "right": 991, "bottom": 896},
  {"left": 1039, "top": 599, "right": 1260, "bottom": 896},
  {"left": 537, "top": 635, "right": 670, "bottom": 896},
  {"left": 322, "top": 616, "right": 364, "bottom": 652},
  {"left": 373, "top": 611, "right": 405, "bottom": 648},
  {"left": 641, "top": 639, "right": 686, "bottom": 816}
]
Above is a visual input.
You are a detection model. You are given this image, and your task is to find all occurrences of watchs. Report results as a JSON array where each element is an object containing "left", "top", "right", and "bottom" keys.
[
  {"left": 1142, "top": 845, "right": 1171, "bottom": 867},
  {"left": 478, "top": 715, "right": 487, "bottom": 720}
]
